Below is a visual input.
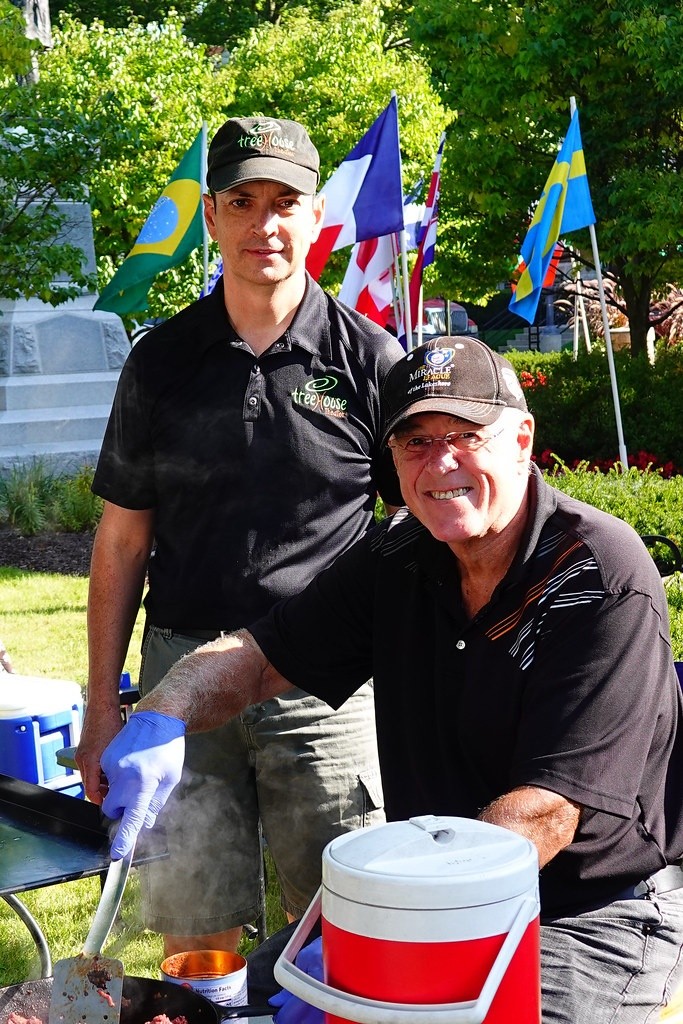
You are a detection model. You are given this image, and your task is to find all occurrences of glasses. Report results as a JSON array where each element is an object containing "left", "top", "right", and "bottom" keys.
[{"left": 385, "top": 421, "right": 515, "bottom": 462}]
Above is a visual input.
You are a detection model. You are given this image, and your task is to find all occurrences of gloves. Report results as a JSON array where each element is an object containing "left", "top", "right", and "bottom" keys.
[
  {"left": 102, "top": 711, "right": 185, "bottom": 859},
  {"left": 269, "top": 935, "right": 325, "bottom": 1024}
]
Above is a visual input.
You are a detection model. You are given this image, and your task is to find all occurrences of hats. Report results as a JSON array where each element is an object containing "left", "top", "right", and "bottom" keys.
[
  {"left": 380, "top": 336, "right": 529, "bottom": 447},
  {"left": 206, "top": 117, "right": 320, "bottom": 195}
]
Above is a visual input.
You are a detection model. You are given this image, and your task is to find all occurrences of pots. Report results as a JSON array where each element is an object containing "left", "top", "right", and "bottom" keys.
[{"left": 0, "top": 976, "right": 281, "bottom": 1024}]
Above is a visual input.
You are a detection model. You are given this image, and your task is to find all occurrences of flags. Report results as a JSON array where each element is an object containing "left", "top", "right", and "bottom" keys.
[
  {"left": 505, "top": 105, "right": 597, "bottom": 324},
  {"left": 90, "top": 129, "right": 210, "bottom": 315},
  {"left": 300, "top": 93, "right": 446, "bottom": 343}
]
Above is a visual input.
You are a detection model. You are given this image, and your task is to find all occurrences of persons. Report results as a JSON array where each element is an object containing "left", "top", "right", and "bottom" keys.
[
  {"left": 101, "top": 337, "right": 682, "bottom": 1022},
  {"left": 75, "top": 115, "right": 419, "bottom": 967}
]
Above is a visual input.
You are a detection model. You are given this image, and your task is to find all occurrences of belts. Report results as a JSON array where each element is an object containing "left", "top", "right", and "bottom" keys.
[
  {"left": 176, "top": 619, "right": 222, "bottom": 641},
  {"left": 617, "top": 862, "right": 683, "bottom": 899}
]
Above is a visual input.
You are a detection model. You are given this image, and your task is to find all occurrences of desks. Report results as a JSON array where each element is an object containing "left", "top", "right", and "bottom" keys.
[{"left": 0, "top": 772, "right": 171, "bottom": 979}]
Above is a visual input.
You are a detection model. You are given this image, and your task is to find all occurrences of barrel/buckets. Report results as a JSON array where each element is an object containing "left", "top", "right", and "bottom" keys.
[
  {"left": 160, "top": 950, "right": 249, "bottom": 1024},
  {"left": 274, "top": 814, "right": 541, "bottom": 1024}
]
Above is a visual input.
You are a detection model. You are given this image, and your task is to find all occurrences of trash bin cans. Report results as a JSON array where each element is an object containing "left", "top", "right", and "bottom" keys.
[{"left": 609, "top": 327, "right": 655, "bottom": 364}]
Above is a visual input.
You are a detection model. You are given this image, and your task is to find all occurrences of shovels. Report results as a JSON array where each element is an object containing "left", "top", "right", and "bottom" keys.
[{"left": 48, "top": 839, "right": 136, "bottom": 1024}]
